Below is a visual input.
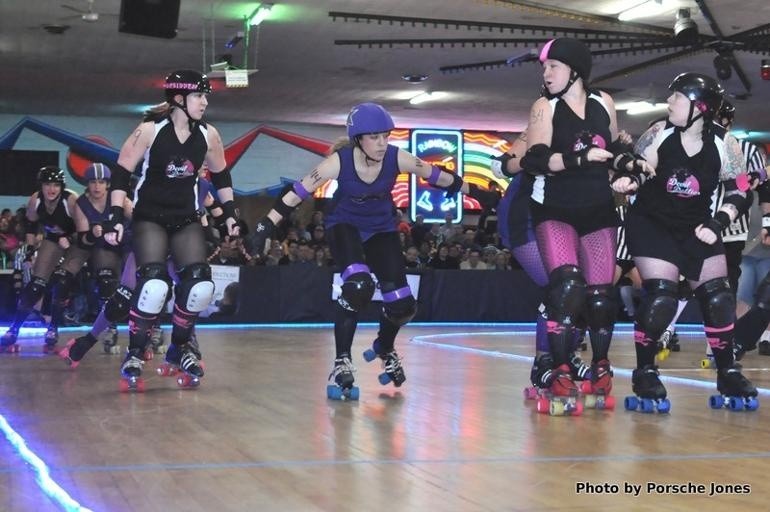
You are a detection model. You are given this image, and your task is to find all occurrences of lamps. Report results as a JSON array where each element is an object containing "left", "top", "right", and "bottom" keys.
[
  {"left": 224, "top": 23, "right": 245, "bottom": 50},
  {"left": 245, "top": 1, "right": 273, "bottom": 26}
]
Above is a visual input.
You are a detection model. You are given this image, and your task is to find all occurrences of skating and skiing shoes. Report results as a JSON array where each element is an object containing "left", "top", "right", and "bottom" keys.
[
  {"left": 538, "top": 363, "right": 585, "bottom": 415},
  {"left": 187, "top": 326, "right": 206, "bottom": 371},
  {"left": 157, "top": 341, "right": 204, "bottom": 388},
  {"left": 624, "top": 366, "right": 670, "bottom": 413},
  {"left": 655, "top": 331, "right": 671, "bottom": 360},
  {"left": 700, "top": 345, "right": 742, "bottom": 368},
  {"left": 327, "top": 353, "right": 359, "bottom": 400},
  {"left": 152, "top": 326, "right": 168, "bottom": 354},
  {"left": 119, "top": 346, "right": 145, "bottom": 392},
  {"left": 524, "top": 354, "right": 553, "bottom": 399},
  {"left": 57, "top": 335, "right": 94, "bottom": 368},
  {"left": 0, "top": 326, "right": 21, "bottom": 353},
  {"left": 568, "top": 350, "right": 592, "bottom": 382},
  {"left": 581, "top": 359, "right": 616, "bottom": 410},
  {"left": 144, "top": 330, "right": 153, "bottom": 361},
  {"left": 42, "top": 325, "right": 60, "bottom": 355},
  {"left": 103, "top": 328, "right": 120, "bottom": 355},
  {"left": 363, "top": 337, "right": 406, "bottom": 387},
  {"left": 709, "top": 365, "right": 759, "bottom": 411},
  {"left": 573, "top": 334, "right": 586, "bottom": 350}
]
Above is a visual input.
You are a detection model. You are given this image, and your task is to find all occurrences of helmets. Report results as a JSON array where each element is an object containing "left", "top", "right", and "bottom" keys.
[
  {"left": 162, "top": 70, "right": 212, "bottom": 103},
  {"left": 719, "top": 100, "right": 736, "bottom": 118},
  {"left": 37, "top": 166, "right": 65, "bottom": 186},
  {"left": 347, "top": 102, "right": 394, "bottom": 138},
  {"left": 539, "top": 37, "right": 592, "bottom": 80},
  {"left": 668, "top": 71, "right": 724, "bottom": 119}
]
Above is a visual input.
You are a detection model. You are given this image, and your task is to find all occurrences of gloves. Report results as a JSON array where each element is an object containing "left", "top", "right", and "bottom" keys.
[
  {"left": 61, "top": 234, "right": 73, "bottom": 245},
  {"left": 254, "top": 216, "right": 275, "bottom": 235},
  {"left": 617, "top": 153, "right": 650, "bottom": 178},
  {"left": 746, "top": 169, "right": 768, "bottom": 184},
  {"left": 762, "top": 212, "right": 770, "bottom": 236},
  {"left": 561, "top": 145, "right": 607, "bottom": 170},
  {"left": 468, "top": 181, "right": 498, "bottom": 210},
  {"left": 23, "top": 244, "right": 34, "bottom": 262},
  {"left": 218, "top": 224, "right": 236, "bottom": 244},
  {"left": 701, "top": 211, "right": 734, "bottom": 238},
  {"left": 610, "top": 173, "right": 642, "bottom": 193},
  {"left": 102, "top": 205, "right": 125, "bottom": 243},
  {"left": 224, "top": 200, "right": 248, "bottom": 236},
  {"left": 86, "top": 227, "right": 98, "bottom": 243}
]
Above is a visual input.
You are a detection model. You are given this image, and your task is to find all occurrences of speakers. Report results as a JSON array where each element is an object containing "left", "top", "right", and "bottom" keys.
[{"left": 117, "top": 0, "right": 181, "bottom": 39}]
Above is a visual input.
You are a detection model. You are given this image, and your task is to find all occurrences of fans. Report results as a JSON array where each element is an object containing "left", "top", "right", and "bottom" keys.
[{"left": 58, "top": 1, "right": 120, "bottom": 23}]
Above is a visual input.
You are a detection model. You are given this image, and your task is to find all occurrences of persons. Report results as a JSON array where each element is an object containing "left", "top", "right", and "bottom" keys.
[
  {"left": 493, "top": 121, "right": 637, "bottom": 389},
  {"left": 236, "top": 101, "right": 504, "bottom": 389},
  {"left": 522, "top": 36, "right": 659, "bottom": 395},
  {"left": 1, "top": 141, "right": 769, "bottom": 405},
  {"left": 99, "top": 68, "right": 251, "bottom": 378},
  {"left": 705, "top": 97, "right": 770, "bottom": 334},
  {"left": 608, "top": 68, "right": 762, "bottom": 403}
]
